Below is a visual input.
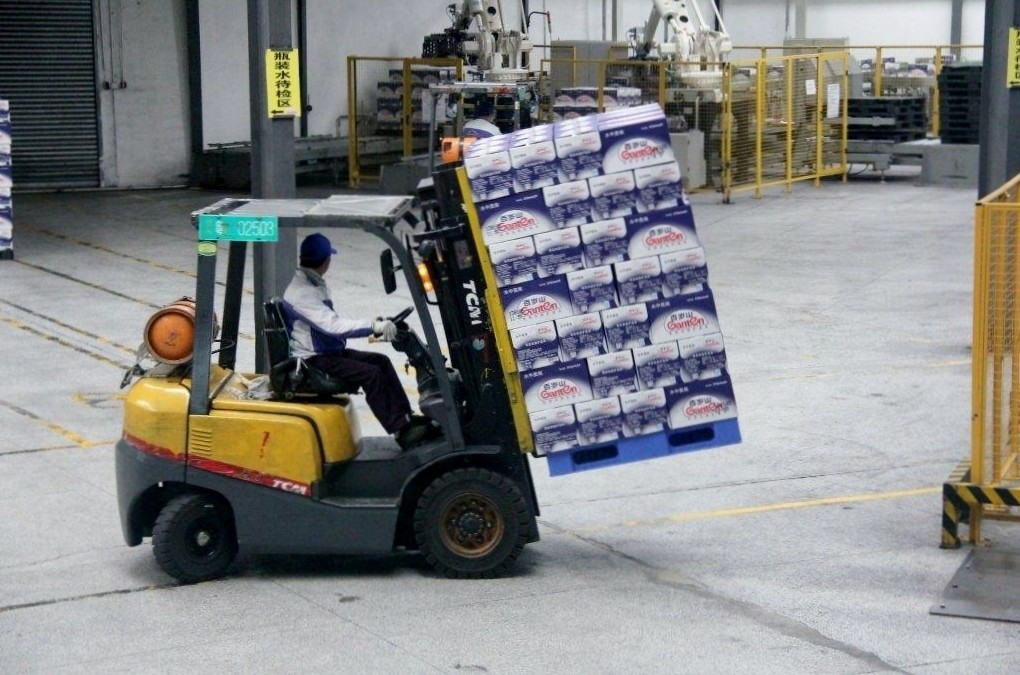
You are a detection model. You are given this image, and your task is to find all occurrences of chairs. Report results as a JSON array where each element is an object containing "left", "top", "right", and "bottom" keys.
[{"left": 260, "top": 298, "right": 360, "bottom": 394}]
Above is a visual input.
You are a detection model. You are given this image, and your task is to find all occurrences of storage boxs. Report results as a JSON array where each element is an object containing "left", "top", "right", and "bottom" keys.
[
  {"left": 378, "top": 35, "right": 739, "bottom": 455},
  {"left": 0, "top": 97, "right": 14, "bottom": 260},
  {"left": 841, "top": 63, "right": 982, "bottom": 144}
]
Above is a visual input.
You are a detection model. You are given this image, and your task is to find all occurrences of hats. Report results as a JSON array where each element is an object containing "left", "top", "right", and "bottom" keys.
[
  {"left": 476, "top": 97, "right": 493, "bottom": 116},
  {"left": 299, "top": 234, "right": 337, "bottom": 256}
]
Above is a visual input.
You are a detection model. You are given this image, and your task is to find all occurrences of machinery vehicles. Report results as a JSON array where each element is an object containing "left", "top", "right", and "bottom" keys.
[{"left": 115, "top": 137, "right": 546, "bottom": 585}]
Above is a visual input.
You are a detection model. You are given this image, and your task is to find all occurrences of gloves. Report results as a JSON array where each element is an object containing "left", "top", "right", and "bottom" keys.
[{"left": 372, "top": 320, "right": 397, "bottom": 343}]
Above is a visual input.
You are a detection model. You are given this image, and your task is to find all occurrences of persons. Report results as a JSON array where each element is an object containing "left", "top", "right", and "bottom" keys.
[
  {"left": 463, "top": 99, "right": 502, "bottom": 140},
  {"left": 281, "top": 233, "right": 434, "bottom": 436}
]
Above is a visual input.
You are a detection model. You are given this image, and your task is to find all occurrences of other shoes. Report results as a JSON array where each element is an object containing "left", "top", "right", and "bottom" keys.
[
  {"left": 410, "top": 413, "right": 432, "bottom": 425},
  {"left": 394, "top": 425, "right": 438, "bottom": 451}
]
